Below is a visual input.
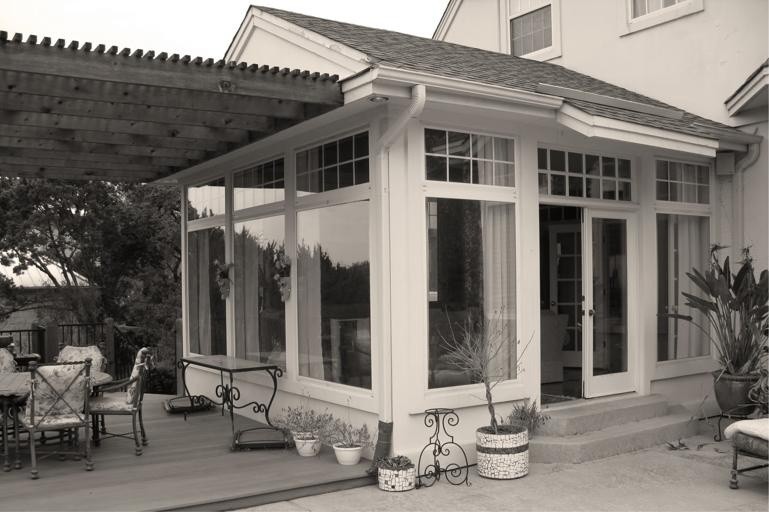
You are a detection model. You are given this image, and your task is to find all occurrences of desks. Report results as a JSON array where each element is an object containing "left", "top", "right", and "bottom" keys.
[{"left": 176, "top": 352, "right": 283, "bottom": 442}]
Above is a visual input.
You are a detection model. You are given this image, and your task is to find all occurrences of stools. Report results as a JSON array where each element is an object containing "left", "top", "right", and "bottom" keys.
[{"left": 720, "top": 417, "right": 769, "bottom": 492}]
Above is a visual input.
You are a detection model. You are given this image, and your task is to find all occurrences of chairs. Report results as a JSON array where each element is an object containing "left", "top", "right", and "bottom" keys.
[{"left": 0, "top": 332, "right": 154, "bottom": 481}]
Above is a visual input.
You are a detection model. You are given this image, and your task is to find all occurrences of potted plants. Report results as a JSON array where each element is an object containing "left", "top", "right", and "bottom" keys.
[
  {"left": 439, "top": 303, "right": 539, "bottom": 482},
  {"left": 505, "top": 398, "right": 550, "bottom": 438},
  {"left": 276, "top": 401, "right": 416, "bottom": 494},
  {"left": 648, "top": 240, "right": 769, "bottom": 419}
]
